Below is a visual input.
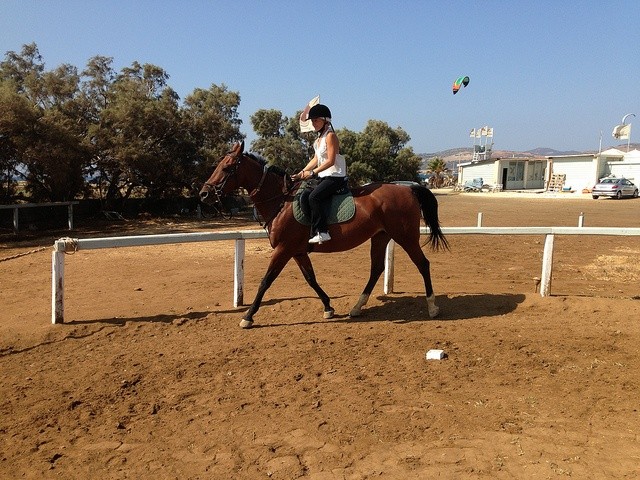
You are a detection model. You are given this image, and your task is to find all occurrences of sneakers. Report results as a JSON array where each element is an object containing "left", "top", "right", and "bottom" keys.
[{"left": 309, "top": 232, "right": 331, "bottom": 243}]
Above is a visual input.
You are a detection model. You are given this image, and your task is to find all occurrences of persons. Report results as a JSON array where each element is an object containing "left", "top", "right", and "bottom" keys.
[{"left": 291, "top": 103, "right": 346, "bottom": 244}]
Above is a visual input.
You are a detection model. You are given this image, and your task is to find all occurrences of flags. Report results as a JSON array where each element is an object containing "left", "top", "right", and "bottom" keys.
[
  {"left": 612, "top": 124, "right": 631, "bottom": 140},
  {"left": 476, "top": 129, "right": 482, "bottom": 137},
  {"left": 487, "top": 128, "right": 493, "bottom": 138},
  {"left": 481, "top": 126, "right": 486, "bottom": 136},
  {"left": 470, "top": 129, "right": 475, "bottom": 137}
]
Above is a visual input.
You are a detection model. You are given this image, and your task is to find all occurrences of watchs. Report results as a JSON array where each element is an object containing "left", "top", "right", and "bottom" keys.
[{"left": 310, "top": 170, "right": 314, "bottom": 177}]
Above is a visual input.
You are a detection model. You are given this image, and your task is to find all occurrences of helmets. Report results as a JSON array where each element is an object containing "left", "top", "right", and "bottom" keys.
[{"left": 305, "top": 104, "right": 331, "bottom": 121}]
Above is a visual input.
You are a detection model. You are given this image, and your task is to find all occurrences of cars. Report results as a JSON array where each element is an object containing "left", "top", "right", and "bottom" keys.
[{"left": 591, "top": 178, "right": 638, "bottom": 200}]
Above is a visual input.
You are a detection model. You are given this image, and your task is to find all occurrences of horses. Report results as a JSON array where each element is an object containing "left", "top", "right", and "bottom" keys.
[{"left": 197, "top": 139, "right": 454, "bottom": 329}]
